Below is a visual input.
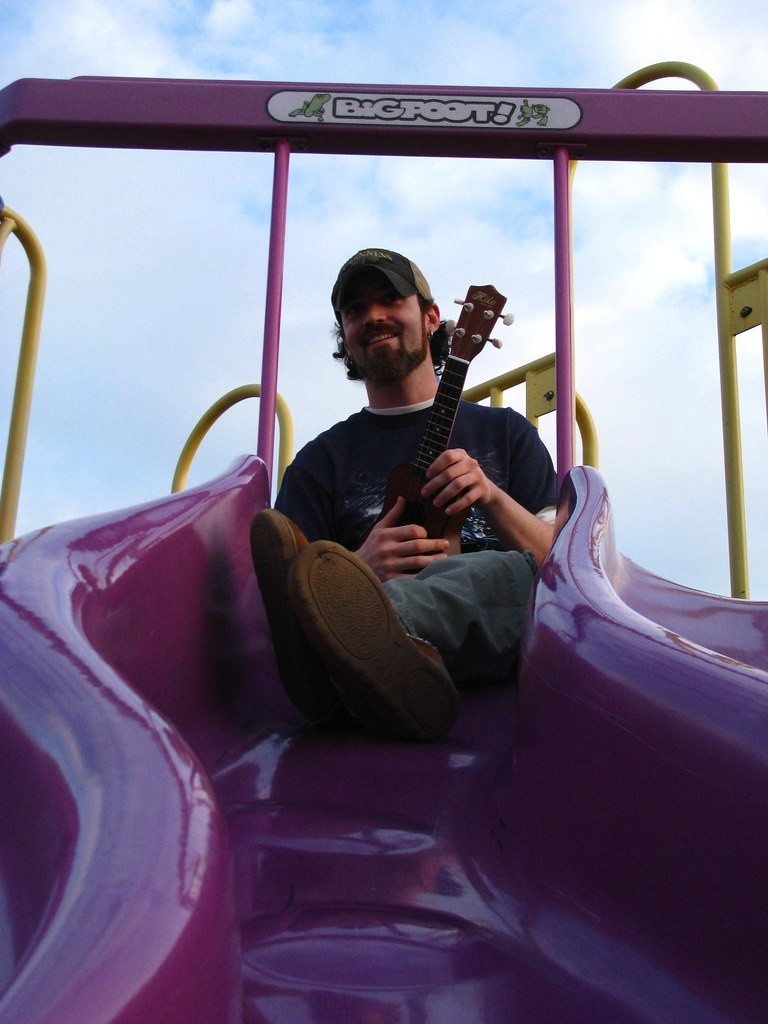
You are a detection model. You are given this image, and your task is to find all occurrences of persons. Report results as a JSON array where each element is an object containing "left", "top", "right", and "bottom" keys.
[{"left": 250, "top": 247, "right": 561, "bottom": 742}]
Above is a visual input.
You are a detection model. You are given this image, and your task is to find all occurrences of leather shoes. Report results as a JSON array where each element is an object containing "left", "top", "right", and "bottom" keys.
[
  {"left": 299, "top": 537, "right": 458, "bottom": 724},
  {"left": 246, "top": 511, "right": 311, "bottom": 664}
]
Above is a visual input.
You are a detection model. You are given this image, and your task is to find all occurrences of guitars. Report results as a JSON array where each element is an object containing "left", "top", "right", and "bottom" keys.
[{"left": 357, "top": 285, "right": 515, "bottom": 555}]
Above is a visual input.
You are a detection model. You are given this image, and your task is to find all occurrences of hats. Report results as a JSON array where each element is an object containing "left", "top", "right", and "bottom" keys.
[{"left": 331, "top": 248, "right": 433, "bottom": 326}]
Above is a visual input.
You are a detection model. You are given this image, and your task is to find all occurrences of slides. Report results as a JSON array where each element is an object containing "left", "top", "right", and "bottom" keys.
[{"left": 0, "top": 448, "right": 768, "bottom": 1024}]
